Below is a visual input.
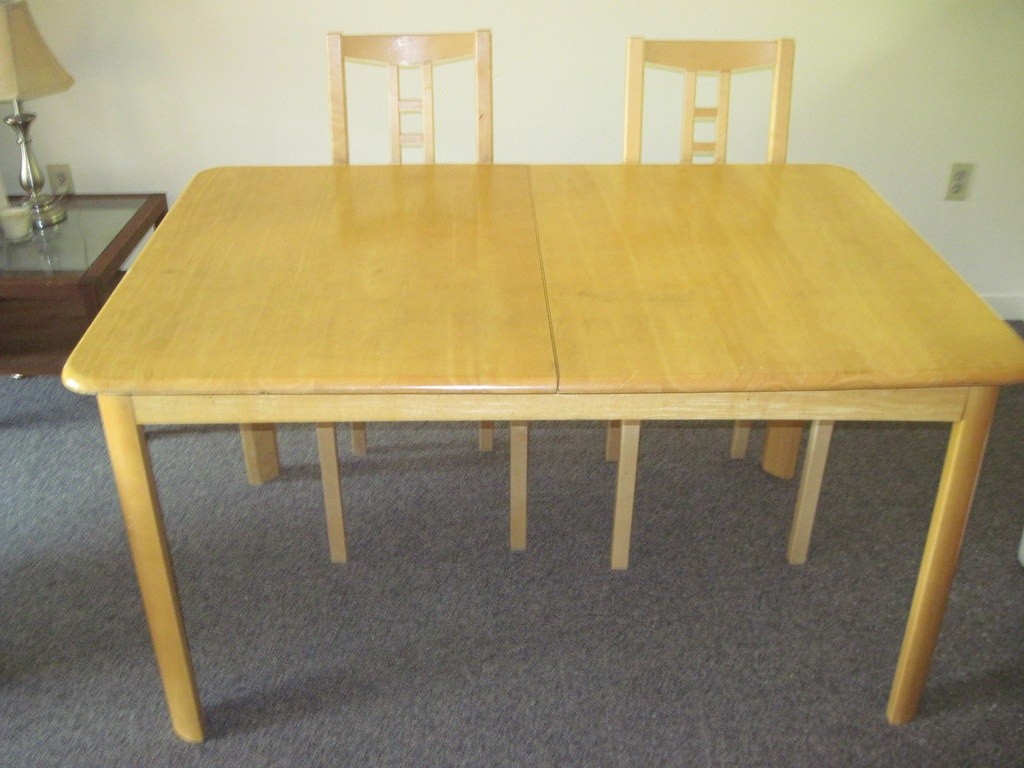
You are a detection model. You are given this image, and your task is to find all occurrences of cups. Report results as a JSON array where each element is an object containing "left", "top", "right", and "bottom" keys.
[
  {"left": 0, "top": 168, "right": 35, "bottom": 244},
  {"left": 47, "top": 162, "right": 75, "bottom": 202}
]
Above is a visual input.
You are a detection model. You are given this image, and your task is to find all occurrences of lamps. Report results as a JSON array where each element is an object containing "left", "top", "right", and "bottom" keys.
[{"left": 0, "top": 0, "right": 82, "bottom": 234}]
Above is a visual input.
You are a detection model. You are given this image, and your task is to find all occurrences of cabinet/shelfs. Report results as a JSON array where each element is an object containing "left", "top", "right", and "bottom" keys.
[{"left": 0, "top": 196, "right": 165, "bottom": 377}]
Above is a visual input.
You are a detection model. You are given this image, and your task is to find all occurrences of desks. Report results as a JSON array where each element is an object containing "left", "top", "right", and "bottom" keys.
[{"left": 76, "top": 158, "right": 1024, "bottom": 746}]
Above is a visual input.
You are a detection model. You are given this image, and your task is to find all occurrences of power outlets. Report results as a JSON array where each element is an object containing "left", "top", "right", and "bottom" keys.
[{"left": 944, "top": 163, "right": 973, "bottom": 203}]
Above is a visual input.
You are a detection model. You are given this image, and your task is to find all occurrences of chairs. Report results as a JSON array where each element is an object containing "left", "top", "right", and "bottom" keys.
[
  {"left": 318, "top": 24, "right": 533, "bottom": 563},
  {"left": 597, "top": 30, "right": 838, "bottom": 573}
]
